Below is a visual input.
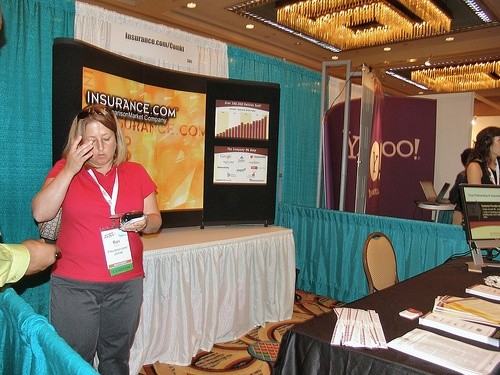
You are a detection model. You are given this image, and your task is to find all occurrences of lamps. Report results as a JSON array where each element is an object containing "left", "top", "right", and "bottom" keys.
[
  {"left": 277, "top": 0, "right": 452, "bottom": 51},
  {"left": 411, "top": 59, "right": 500, "bottom": 91}
]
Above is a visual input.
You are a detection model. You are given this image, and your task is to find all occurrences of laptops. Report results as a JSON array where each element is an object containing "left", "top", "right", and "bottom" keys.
[
  {"left": 421, "top": 182, "right": 450, "bottom": 204},
  {"left": 419, "top": 181, "right": 450, "bottom": 203}
]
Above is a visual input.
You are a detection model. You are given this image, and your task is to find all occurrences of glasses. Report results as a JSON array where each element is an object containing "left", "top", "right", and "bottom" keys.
[{"left": 77, "top": 107, "right": 110, "bottom": 129}]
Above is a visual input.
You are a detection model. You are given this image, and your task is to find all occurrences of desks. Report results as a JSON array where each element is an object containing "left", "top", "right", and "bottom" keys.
[
  {"left": 92, "top": 224, "right": 296, "bottom": 375},
  {"left": 418, "top": 202, "right": 455, "bottom": 223},
  {"left": 272, "top": 255, "right": 500, "bottom": 375}
]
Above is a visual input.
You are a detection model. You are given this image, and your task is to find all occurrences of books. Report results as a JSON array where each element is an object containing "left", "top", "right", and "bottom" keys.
[
  {"left": 465, "top": 284, "right": 500, "bottom": 301},
  {"left": 418, "top": 295, "right": 500, "bottom": 348}
]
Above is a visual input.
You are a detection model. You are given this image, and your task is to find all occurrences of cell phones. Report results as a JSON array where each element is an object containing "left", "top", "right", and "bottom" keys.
[{"left": 121, "top": 210, "right": 143, "bottom": 225}]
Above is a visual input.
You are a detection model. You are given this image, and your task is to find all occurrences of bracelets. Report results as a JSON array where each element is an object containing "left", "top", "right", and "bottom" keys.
[{"left": 140, "top": 221, "right": 148, "bottom": 232}]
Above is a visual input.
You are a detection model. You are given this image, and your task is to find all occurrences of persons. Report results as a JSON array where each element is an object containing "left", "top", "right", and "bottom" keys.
[
  {"left": 33, "top": 103, "right": 161, "bottom": 375},
  {"left": 453, "top": 126, "right": 500, "bottom": 226},
  {"left": 0, "top": 239, "right": 60, "bottom": 288}
]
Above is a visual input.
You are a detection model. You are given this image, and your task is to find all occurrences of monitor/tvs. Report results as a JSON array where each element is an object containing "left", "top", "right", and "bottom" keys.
[{"left": 459, "top": 183, "right": 500, "bottom": 268}]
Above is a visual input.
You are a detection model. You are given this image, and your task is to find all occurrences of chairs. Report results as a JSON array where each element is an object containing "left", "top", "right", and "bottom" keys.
[{"left": 364, "top": 232, "right": 400, "bottom": 294}]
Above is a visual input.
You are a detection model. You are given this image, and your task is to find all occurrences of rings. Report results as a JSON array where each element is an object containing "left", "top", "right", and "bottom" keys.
[{"left": 135, "top": 229, "right": 138, "bottom": 233}]
